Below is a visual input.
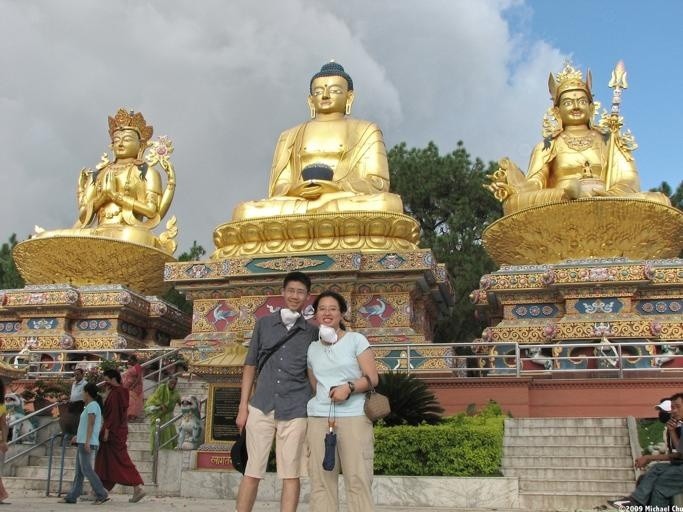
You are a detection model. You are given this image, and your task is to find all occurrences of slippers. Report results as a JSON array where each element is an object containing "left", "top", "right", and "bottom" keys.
[
  {"left": 91, "top": 498, "right": 111, "bottom": 505},
  {"left": 57, "top": 498, "right": 76, "bottom": 503}
]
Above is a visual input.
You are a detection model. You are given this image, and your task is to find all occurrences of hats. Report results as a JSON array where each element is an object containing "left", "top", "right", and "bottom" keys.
[
  {"left": 654, "top": 400, "right": 671, "bottom": 412},
  {"left": 230, "top": 430, "right": 247, "bottom": 476}
]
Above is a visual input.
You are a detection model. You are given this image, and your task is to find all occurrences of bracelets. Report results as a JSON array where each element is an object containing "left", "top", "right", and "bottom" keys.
[{"left": 110, "top": 192, "right": 120, "bottom": 202}]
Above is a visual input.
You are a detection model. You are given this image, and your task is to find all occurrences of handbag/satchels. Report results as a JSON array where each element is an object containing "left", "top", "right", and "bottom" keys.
[
  {"left": 362, "top": 374, "right": 390, "bottom": 422},
  {"left": 94, "top": 441, "right": 113, "bottom": 481}
]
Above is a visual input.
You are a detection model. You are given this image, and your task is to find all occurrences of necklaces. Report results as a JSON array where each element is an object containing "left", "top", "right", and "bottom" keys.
[
  {"left": 558, "top": 130, "right": 597, "bottom": 151},
  {"left": 96, "top": 162, "right": 133, "bottom": 219}
]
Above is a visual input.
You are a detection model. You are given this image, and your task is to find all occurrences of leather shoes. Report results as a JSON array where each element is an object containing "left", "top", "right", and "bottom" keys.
[{"left": 607, "top": 497, "right": 631, "bottom": 509}]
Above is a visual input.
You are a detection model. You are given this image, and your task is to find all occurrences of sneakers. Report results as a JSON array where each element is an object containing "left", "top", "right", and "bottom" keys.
[
  {"left": 614, "top": 501, "right": 639, "bottom": 512},
  {"left": 128, "top": 488, "right": 146, "bottom": 502}
]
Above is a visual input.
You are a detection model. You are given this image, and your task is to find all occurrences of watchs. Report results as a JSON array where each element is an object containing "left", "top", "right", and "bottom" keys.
[{"left": 347, "top": 381, "right": 355, "bottom": 395}]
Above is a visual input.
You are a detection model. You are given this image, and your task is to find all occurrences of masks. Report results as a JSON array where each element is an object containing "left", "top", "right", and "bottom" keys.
[
  {"left": 319, "top": 325, "right": 337, "bottom": 344},
  {"left": 280, "top": 309, "right": 300, "bottom": 331}
]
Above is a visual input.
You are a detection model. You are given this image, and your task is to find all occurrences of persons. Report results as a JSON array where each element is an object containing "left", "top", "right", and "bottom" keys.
[
  {"left": 27, "top": 110, "right": 164, "bottom": 253},
  {"left": 225, "top": 60, "right": 406, "bottom": 220},
  {"left": 303, "top": 289, "right": 382, "bottom": 510},
  {"left": 0, "top": 379, "right": 11, "bottom": 504},
  {"left": 98, "top": 369, "right": 151, "bottom": 501},
  {"left": 117, "top": 354, "right": 145, "bottom": 420},
  {"left": 575, "top": 157, "right": 594, "bottom": 178},
  {"left": 232, "top": 273, "right": 316, "bottom": 511},
  {"left": 68, "top": 368, "right": 87, "bottom": 403},
  {"left": 58, "top": 383, "right": 109, "bottom": 504},
  {"left": 480, "top": 65, "right": 672, "bottom": 212},
  {"left": 651, "top": 399, "right": 674, "bottom": 453},
  {"left": 144, "top": 374, "right": 179, "bottom": 451},
  {"left": 606, "top": 391, "right": 683, "bottom": 512}
]
